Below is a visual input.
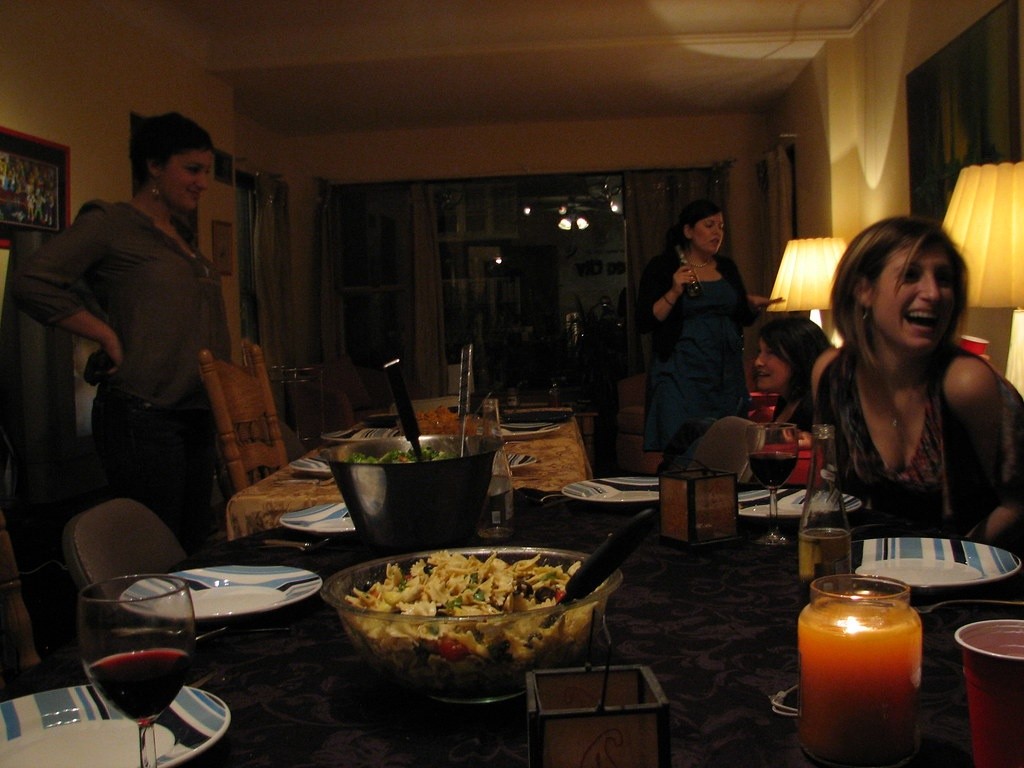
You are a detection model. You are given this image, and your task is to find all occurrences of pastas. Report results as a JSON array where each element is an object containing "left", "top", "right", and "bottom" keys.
[{"left": 338, "top": 549, "right": 607, "bottom": 698}]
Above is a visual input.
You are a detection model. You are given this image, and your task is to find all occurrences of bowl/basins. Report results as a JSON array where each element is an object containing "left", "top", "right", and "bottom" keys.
[
  {"left": 319, "top": 545, "right": 624, "bottom": 703},
  {"left": 320, "top": 434, "right": 505, "bottom": 560}
]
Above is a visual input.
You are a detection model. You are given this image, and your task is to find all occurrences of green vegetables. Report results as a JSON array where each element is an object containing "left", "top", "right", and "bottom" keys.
[
  {"left": 344, "top": 447, "right": 456, "bottom": 463},
  {"left": 398, "top": 571, "right": 569, "bottom": 659}
]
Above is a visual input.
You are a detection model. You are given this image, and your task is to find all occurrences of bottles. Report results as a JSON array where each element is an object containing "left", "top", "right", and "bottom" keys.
[
  {"left": 549, "top": 383, "right": 560, "bottom": 407},
  {"left": 476, "top": 399, "right": 514, "bottom": 539},
  {"left": 798, "top": 424, "right": 852, "bottom": 593},
  {"left": 676, "top": 244, "right": 704, "bottom": 297}
]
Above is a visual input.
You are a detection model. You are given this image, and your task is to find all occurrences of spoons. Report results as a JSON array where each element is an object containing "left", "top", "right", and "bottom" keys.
[{"left": 525, "top": 494, "right": 565, "bottom": 505}]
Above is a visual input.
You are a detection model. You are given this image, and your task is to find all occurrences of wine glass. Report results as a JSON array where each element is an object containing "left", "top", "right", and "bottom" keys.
[
  {"left": 79, "top": 573, "right": 197, "bottom": 768},
  {"left": 746, "top": 422, "right": 799, "bottom": 547}
]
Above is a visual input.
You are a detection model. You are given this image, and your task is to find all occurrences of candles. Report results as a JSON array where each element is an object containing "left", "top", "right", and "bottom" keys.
[{"left": 797, "top": 572, "right": 923, "bottom": 768}]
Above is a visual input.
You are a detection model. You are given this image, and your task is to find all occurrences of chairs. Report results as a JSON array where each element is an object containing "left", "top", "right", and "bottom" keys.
[
  {"left": 663, "top": 415, "right": 762, "bottom": 481},
  {"left": 63, "top": 496, "right": 190, "bottom": 610},
  {"left": 199, "top": 333, "right": 292, "bottom": 490}
]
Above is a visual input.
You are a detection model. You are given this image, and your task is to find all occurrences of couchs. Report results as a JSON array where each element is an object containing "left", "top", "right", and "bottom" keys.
[{"left": 616, "top": 370, "right": 650, "bottom": 466}]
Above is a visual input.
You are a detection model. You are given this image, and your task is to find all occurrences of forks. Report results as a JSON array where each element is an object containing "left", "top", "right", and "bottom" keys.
[
  {"left": 914, "top": 599, "right": 1024, "bottom": 613},
  {"left": 259, "top": 536, "right": 331, "bottom": 550},
  {"left": 113, "top": 627, "right": 227, "bottom": 640}
]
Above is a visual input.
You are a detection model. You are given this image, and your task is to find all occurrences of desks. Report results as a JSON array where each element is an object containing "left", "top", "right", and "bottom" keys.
[
  {"left": 1, "top": 456, "right": 1024, "bottom": 768},
  {"left": 226, "top": 392, "right": 591, "bottom": 549}
]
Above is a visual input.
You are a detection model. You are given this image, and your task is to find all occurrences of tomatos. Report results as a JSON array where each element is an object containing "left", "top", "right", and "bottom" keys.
[{"left": 440, "top": 636, "right": 466, "bottom": 661}]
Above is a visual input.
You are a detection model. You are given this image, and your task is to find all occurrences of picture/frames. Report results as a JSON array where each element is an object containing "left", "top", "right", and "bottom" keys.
[
  {"left": 0, "top": 126, "right": 73, "bottom": 252},
  {"left": 210, "top": 220, "right": 236, "bottom": 273}
]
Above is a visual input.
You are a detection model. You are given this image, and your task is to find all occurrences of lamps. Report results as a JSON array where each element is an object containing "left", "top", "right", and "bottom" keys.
[
  {"left": 765, "top": 236, "right": 847, "bottom": 348},
  {"left": 521, "top": 178, "right": 626, "bottom": 232},
  {"left": 923, "top": 161, "right": 1024, "bottom": 388}
]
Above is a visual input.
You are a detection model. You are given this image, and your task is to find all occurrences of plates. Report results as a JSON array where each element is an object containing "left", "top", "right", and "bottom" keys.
[
  {"left": 0, "top": 685, "right": 232, "bottom": 768},
  {"left": 321, "top": 428, "right": 400, "bottom": 444},
  {"left": 280, "top": 501, "right": 357, "bottom": 534},
  {"left": 506, "top": 453, "right": 538, "bottom": 470},
  {"left": 120, "top": 565, "right": 323, "bottom": 618},
  {"left": 289, "top": 454, "right": 334, "bottom": 476},
  {"left": 849, "top": 537, "right": 1022, "bottom": 588},
  {"left": 499, "top": 422, "right": 560, "bottom": 440},
  {"left": 738, "top": 489, "right": 863, "bottom": 519},
  {"left": 561, "top": 476, "right": 662, "bottom": 502}
]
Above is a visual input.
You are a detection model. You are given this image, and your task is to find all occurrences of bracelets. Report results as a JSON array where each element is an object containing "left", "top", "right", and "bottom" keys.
[{"left": 664, "top": 296, "right": 674, "bottom": 307}]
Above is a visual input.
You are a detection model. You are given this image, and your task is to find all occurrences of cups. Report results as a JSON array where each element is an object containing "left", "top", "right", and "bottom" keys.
[{"left": 954, "top": 619, "right": 1024, "bottom": 768}]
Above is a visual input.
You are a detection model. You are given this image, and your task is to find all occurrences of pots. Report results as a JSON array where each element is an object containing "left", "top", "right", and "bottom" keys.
[{"left": 504, "top": 411, "right": 598, "bottom": 424}]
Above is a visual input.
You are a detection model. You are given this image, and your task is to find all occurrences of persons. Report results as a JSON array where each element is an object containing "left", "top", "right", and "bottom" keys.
[
  {"left": 754, "top": 317, "right": 830, "bottom": 450},
  {"left": 813, "top": 217, "right": 1024, "bottom": 551},
  {"left": 633, "top": 200, "right": 783, "bottom": 474},
  {"left": 11, "top": 112, "right": 233, "bottom": 555}
]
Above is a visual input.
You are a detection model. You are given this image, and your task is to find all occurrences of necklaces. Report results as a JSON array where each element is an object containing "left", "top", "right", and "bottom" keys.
[
  {"left": 871, "top": 382, "right": 916, "bottom": 427},
  {"left": 687, "top": 253, "right": 711, "bottom": 267}
]
